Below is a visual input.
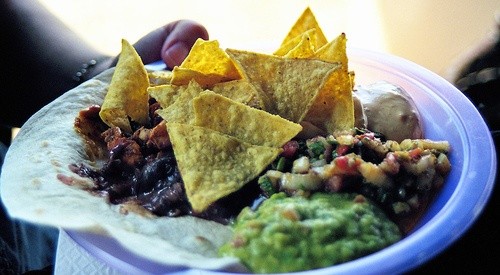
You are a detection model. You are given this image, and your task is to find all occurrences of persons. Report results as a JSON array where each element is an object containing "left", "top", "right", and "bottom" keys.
[{"left": 0, "top": 0, "right": 209, "bottom": 275}]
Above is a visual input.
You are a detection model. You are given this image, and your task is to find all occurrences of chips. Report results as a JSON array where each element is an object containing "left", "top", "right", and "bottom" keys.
[{"left": 97, "top": 6, "right": 354, "bottom": 212}]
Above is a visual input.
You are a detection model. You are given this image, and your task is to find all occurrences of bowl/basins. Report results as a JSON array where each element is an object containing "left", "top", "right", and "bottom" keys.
[{"left": 56, "top": 48, "right": 497, "bottom": 275}]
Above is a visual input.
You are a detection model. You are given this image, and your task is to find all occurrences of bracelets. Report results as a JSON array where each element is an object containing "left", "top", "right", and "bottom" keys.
[{"left": 69, "top": 54, "right": 108, "bottom": 88}]
